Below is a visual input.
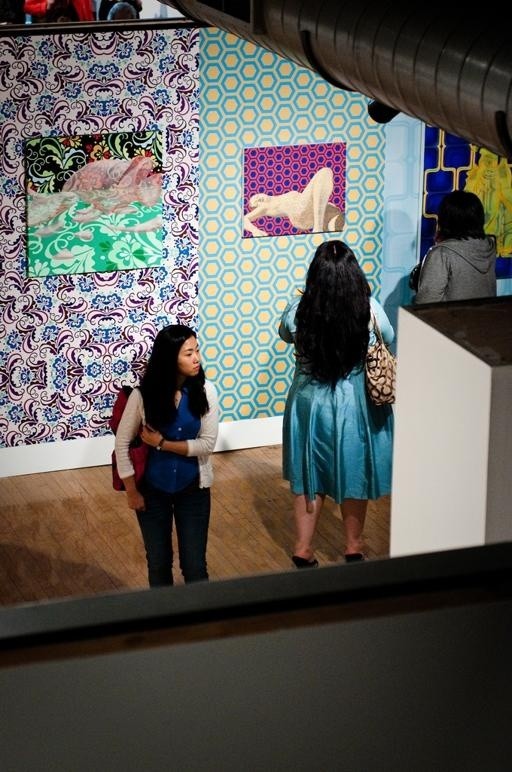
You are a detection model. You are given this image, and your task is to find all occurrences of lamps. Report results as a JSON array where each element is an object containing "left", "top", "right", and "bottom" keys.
[{"left": 368, "top": 99, "right": 399, "bottom": 123}]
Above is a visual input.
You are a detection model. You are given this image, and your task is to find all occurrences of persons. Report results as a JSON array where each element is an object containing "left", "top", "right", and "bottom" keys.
[
  {"left": 241, "top": 166, "right": 347, "bottom": 239},
  {"left": 113, "top": 323, "right": 221, "bottom": 588},
  {"left": 410, "top": 189, "right": 498, "bottom": 306},
  {"left": 277, "top": 239, "right": 395, "bottom": 570}
]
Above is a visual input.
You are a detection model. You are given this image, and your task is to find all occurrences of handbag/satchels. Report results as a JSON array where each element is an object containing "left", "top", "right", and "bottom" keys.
[
  {"left": 366, "top": 341, "right": 397, "bottom": 406},
  {"left": 110, "top": 386, "right": 149, "bottom": 490}
]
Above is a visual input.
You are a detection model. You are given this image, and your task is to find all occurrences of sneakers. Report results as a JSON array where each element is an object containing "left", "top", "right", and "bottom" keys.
[
  {"left": 345, "top": 551, "right": 365, "bottom": 563},
  {"left": 292, "top": 555, "right": 318, "bottom": 568}
]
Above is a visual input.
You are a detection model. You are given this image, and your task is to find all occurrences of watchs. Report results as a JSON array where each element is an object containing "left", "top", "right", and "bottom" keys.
[{"left": 155, "top": 438, "right": 165, "bottom": 451}]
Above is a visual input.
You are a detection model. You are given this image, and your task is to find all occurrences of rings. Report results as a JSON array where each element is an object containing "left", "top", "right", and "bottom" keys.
[{"left": 141, "top": 433, "right": 146, "bottom": 438}]
